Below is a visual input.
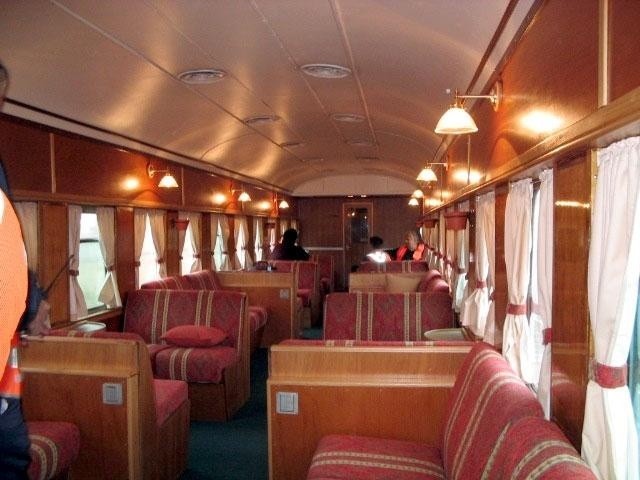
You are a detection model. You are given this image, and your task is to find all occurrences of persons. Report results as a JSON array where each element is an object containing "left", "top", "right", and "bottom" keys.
[
  {"left": 1, "top": 61, "right": 50, "bottom": 480},
  {"left": 386, "top": 229, "right": 426, "bottom": 261},
  {"left": 351, "top": 235, "right": 391, "bottom": 267},
  {"left": 268, "top": 228, "right": 310, "bottom": 261}
]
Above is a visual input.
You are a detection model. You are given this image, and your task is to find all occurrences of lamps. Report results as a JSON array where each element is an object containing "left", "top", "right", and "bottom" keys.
[
  {"left": 416, "top": 161, "right": 448, "bottom": 181},
  {"left": 410, "top": 186, "right": 431, "bottom": 198},
  {"left": 274, "top": 197, "right": 289, "bottom": 208},
  {"left": 230, "top": 185, "right": 252, "bottom": 201},
  {"left": 147, "top": 164, "right": 179, "bottom": 188},
  {"left": 434, "top": 82, "right": 502, "bottom": 135},
  {"left": 408, "top": 199, "right": 419, "bottom": 205}
]
